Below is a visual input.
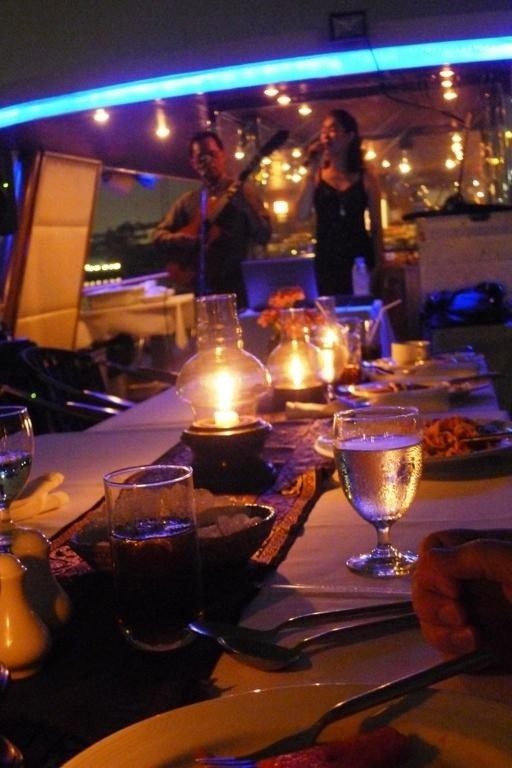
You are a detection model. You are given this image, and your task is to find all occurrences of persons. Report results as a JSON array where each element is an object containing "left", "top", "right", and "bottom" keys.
[
  {"left": 150, "top": 131, "right": 271, "bottom": 312},
  {"left": 294, "top": 107, "right": 382, "bottom": 296}
]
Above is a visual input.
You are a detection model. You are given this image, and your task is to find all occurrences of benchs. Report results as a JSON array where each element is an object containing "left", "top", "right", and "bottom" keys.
[{"left": 432, "top": 322, "right": 512, "bottom": 406}]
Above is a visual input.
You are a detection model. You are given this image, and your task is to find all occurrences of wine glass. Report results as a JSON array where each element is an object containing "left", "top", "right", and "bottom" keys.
[
  {"left": 331, "top": 406, "right": 425, "bottom": 579},
  {"left": 0, "top": 405, "right": 35, "bottom": 554}
]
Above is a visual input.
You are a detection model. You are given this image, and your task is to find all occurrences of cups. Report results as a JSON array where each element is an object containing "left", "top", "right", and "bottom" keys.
[
  {"left": 391, "top": 339, "right": 431, "bottom": 368},
  {"left": 103, "top": 464, "right": 205, "bottom": 652}
]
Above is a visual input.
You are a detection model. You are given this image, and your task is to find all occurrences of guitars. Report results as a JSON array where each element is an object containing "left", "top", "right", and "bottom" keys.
[{"left": 160, "top": 129, "right": 290, "bottom": 290}]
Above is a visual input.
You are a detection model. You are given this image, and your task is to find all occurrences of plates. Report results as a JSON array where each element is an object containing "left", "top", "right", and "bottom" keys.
[
  {"left": 346, "top": 376, "right": 490, "bottom": 405},
  {"left": 59, "top": 681, "right": 511, "bottom": 768},
  {"left": 67, "top": 502, "right": 277, "bottom": 579},
  {"left": 314, "top": 416, "right": 511, "bottom": 467}
]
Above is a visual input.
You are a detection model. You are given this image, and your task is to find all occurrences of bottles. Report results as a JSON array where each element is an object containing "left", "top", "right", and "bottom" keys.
[
  {"left": 351, "top": 257, "right": 369, "bottom": 297},
  {"left": 0, "top": 529, "right": 69, "bottom": 679}
]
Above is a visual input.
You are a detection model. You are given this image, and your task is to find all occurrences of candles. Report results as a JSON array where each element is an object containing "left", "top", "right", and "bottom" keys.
[{"left": 213, "top": 406, "right": 239, "bottom": 428}]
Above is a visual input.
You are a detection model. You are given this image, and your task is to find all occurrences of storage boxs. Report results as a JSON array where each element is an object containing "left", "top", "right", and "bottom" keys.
[{"left": 240, "top": 256, "right": 320, "bottom": 311}]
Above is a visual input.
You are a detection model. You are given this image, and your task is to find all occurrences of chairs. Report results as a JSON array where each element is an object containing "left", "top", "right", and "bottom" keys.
[{"left": 0, "top": 347, "right": 180, "bottom": 431}]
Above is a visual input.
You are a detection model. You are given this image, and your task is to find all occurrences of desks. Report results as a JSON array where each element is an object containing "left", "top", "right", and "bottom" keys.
[
  {"left": 242, "top": 303, "right": 422, "bottom": 359},
  {"left": 84, "top": 348, "right": 498, "bottom": 432},
  {"left": 0, "top": 431, "right": 511, "bottom": 766},
  {"left": 76, "top": 293, "right": 193, "bottom": 388}
]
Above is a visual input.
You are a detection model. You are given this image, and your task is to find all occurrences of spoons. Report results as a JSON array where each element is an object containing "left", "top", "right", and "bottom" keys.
[
  {"left": 217, "top": 614, "right": 420, "bottom": 673},
  {"left": 189, "top": 599, "right": 419, "bottom": 645}
]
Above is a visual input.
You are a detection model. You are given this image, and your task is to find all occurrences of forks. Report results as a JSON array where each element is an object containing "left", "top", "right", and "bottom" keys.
[{"left": 195, "top": 651, "right": 498, "bottom": 768}]
